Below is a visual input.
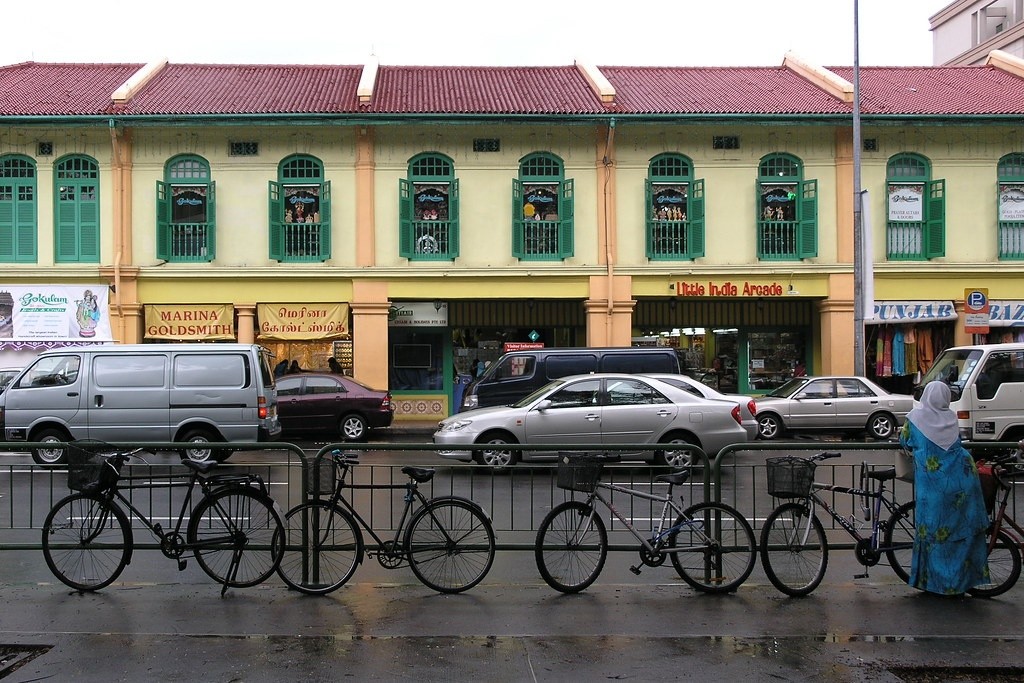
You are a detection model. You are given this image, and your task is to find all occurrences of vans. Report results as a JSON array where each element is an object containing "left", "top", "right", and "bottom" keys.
[
  {"left": 0, "top": 341, "right": 280, "bottom": 469},
  {"left": 458, "top": 346, "right": 682, "bottom": 415}
]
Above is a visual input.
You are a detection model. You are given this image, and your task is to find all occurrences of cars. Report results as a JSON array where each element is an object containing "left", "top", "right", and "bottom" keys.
[
  {"left": 751, "top": 374, "right": 914, "bottom": 442},
  {"left": 433, "top": 371, "right": 748, "bottom": 475},
  {"left": 272, "top": 373, "right": 395, "bottom": 440},
  {"left": 633, "top": 370, "right": 759, "bottom": 440}
]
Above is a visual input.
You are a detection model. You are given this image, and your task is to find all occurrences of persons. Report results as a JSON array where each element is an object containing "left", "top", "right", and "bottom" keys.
[
  {"left": 327, "top": 357, "right": 343, "bottom": 374},
  {"left": 287, "top": 360, "right": 302, "bottom": 375},
  {"left": 898, "top": 381, "right": 991, "bottom": 597},
  {"left": 273, "top": 359, "right": 289, "bottom": 378}
]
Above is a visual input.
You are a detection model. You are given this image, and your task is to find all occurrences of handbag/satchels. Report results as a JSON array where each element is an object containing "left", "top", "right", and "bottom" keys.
[{"left": 894, "top": 450, "right": 916, "bottom": 484}]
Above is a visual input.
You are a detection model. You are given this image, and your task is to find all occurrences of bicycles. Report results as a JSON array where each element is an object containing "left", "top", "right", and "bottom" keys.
[
  {"left": 40, "top": 435, "right": 288, "bottom": 597},
  {"left": 271, "top": 447, "right": 497, "bottom": 594},
  {"left": 534, "top": 448, "right": 756, "bottom": 596},
  {"left": 760, "top": 451, "right": 915, "bottom": 599},
  {"left": 966, "top": 440, "right": 1023, "bottom": 598}
]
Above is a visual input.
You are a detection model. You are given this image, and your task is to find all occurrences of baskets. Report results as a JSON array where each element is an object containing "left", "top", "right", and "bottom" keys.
[
  {"left": 556, "top": 450, "right": 607, "bottom": 493},
  {"left": 978, "top": 472, "right": 1000, "bottom": 513},
  {"left": 765, "top": 457, "right": 817, "bottom": 499},
  {"left": 305, "top": 458, "right": 336, "bottom": 494},
  {"left": 67, "top": 439, "right": 114, "bottom": 492}
]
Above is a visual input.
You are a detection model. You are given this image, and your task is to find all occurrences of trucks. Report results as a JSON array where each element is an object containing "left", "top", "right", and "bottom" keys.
[{"left": 912, "top": 342, "right": 1024, "bottom": 472}]
